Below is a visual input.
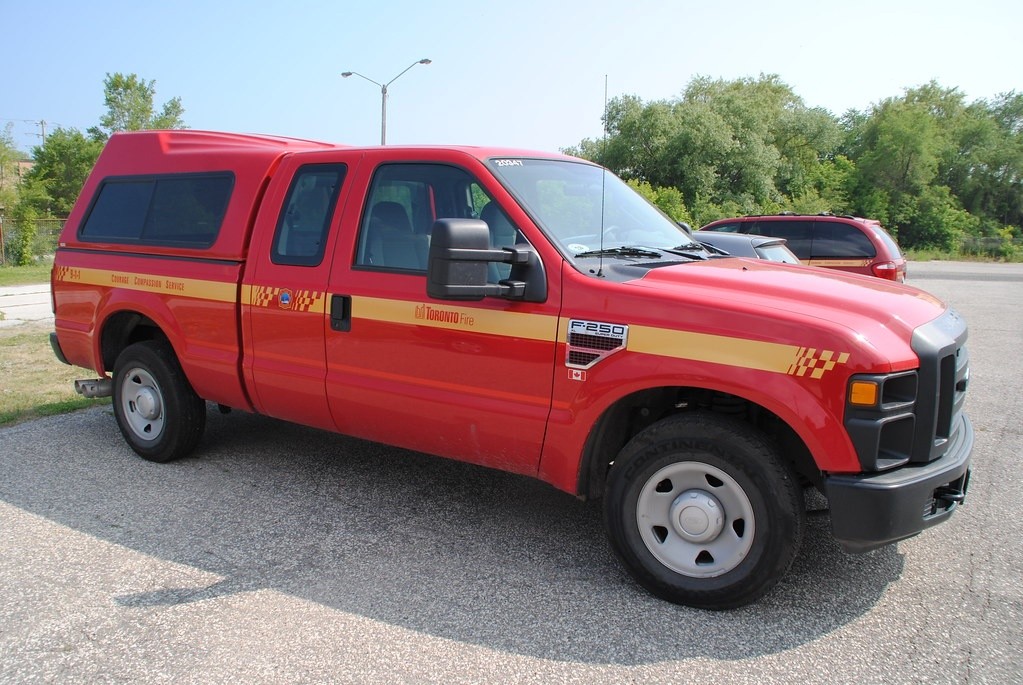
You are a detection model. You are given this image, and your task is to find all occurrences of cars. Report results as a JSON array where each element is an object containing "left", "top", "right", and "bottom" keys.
[{"left": 560, "top": 225, "right": 800, "bottom": 268}]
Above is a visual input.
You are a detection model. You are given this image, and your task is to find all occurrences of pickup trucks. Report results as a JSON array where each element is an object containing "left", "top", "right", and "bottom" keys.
[{"left": 50, "top": 129, "right": 972, "bottom": 612}]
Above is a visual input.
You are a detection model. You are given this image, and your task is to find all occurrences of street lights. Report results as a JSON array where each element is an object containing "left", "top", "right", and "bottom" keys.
[{"left": 339, "top": 56, "right": 432, "bottom": 146}]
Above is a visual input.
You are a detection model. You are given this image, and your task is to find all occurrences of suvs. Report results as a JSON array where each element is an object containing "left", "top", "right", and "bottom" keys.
[{"left": 698, "top": 212, "right": 907, "bottom": 288}]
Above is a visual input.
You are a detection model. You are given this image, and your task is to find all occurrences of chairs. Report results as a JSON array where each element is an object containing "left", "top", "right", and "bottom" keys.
[
  {"left": 366, "top": 201, "right": 430, "bottom": 271},
  {"left": 480, "top": 201, "right": 518, "bottom": 285}
]
